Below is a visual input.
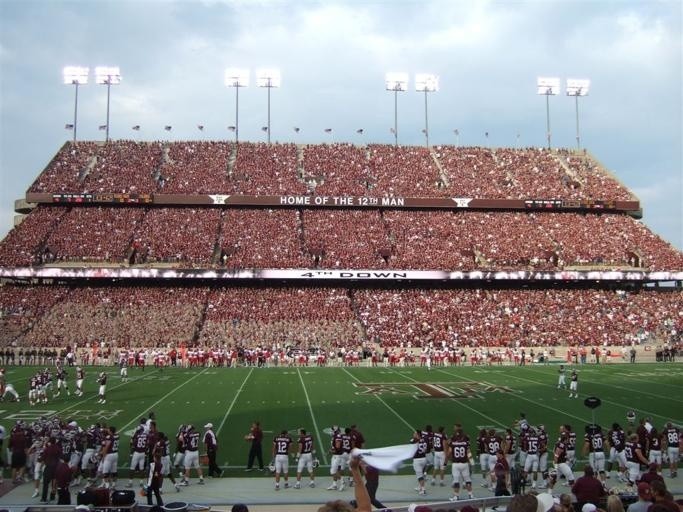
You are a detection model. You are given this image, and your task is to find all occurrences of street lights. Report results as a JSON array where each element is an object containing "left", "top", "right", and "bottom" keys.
[
  {"left": 565, "top": 77, "right": 590, "bottom": 149},
  {"left": 63, "top": 65, "right": 90, "bottom": 149},
  {"left": 414, "top": 71, "right": 439, "bottom": 148},
  {"left": 256, "top": 68, "right": 281, "bottom": 145},
  {"left": 226, "top": 67, "right": 250, "bottom": 144},
  {"left": 385, "top": 68, "right": 409, "bottom": 145},
  {"left": 94, "top": 65, "right": 121, "bottom": 144},
  {"left": 536, "top": 75, "right": 559, "bottom": 149}
]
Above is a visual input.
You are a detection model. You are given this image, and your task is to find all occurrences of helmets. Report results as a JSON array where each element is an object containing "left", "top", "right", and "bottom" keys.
[
  {"left": 520, "top": 423, "right": 529, "bottom": 431},
  {"left": 11, "top": 415, "right": 98, "bottom": 449}
]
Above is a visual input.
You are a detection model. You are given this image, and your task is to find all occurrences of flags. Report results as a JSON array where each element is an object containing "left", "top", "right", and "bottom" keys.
[{"left": 67, "top": 124, "right": 488, "bottom": 135}]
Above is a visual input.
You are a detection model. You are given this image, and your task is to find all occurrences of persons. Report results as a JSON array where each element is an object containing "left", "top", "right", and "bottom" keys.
[{"left": 0, "top": 142, "right": 683, "bottom": 512}]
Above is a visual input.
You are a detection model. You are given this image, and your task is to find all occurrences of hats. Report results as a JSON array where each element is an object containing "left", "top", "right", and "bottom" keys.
[{"left": 204, "top": 423, "right": 213, "bottom": 429}]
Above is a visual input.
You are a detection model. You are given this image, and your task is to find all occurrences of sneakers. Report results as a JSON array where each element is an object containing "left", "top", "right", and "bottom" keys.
[
  {"left": 243, "top": 466, "right": 356, "bottom": 492},
  {"left": 0, "top": 468, "right": 225, "bottom": 504},
  {"left": 28, "top": 390, "right": 107, "bottom": 406},
  {"left": 415, "top": 469, "right": 678, "bottom": 500}
]
